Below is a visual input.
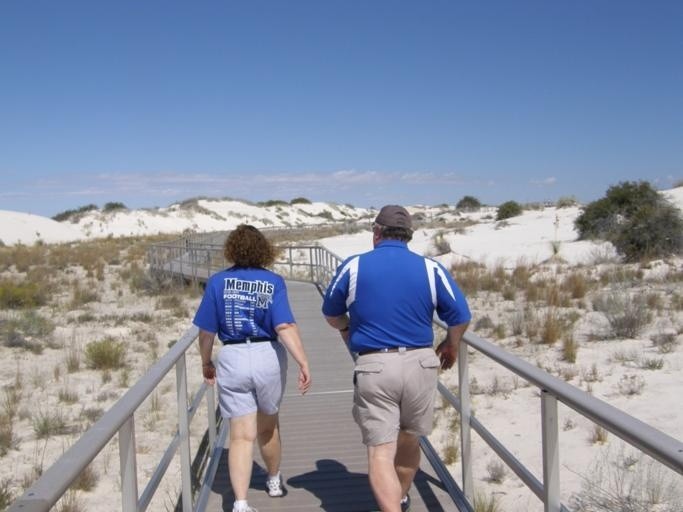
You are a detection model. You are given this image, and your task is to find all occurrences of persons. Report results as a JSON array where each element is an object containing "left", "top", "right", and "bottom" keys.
[
  {"left": 191, "top": 223, "right": 311, "bottom": 511},
  {"left": 320, "top": 204, "right": 472, "bottom": 512}
]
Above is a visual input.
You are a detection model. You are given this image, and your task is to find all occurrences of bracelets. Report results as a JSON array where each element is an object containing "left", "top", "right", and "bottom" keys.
[
  {"left": 339, "top": 324, "right": 349, "bottom": 333},
  {"left": 201, "top": 360, "right": 213, "bottom": 366}
]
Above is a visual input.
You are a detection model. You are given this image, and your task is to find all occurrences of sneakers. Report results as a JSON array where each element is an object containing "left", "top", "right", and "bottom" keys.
[
  {"left": 400, "top": 492, "right": 411, "bottom": 512},
  {"left": 265, "top": 477, "right": 284, "bottom": 497},
  {"left": 233, "top": 503, "right": 258, "bottom": 512}
]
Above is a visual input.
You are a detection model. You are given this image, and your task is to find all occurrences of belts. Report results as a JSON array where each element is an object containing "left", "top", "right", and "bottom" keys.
[
  {"left": 359, "top": 347, "right": 431, "bottom": 355},
  {"left": 224, "top": 337, "right": 277, "bottom": 344}
]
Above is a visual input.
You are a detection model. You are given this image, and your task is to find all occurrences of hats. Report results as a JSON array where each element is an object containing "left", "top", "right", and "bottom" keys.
[{"left": 375, "top": 205, "right": 412, "bottom": 229}]
